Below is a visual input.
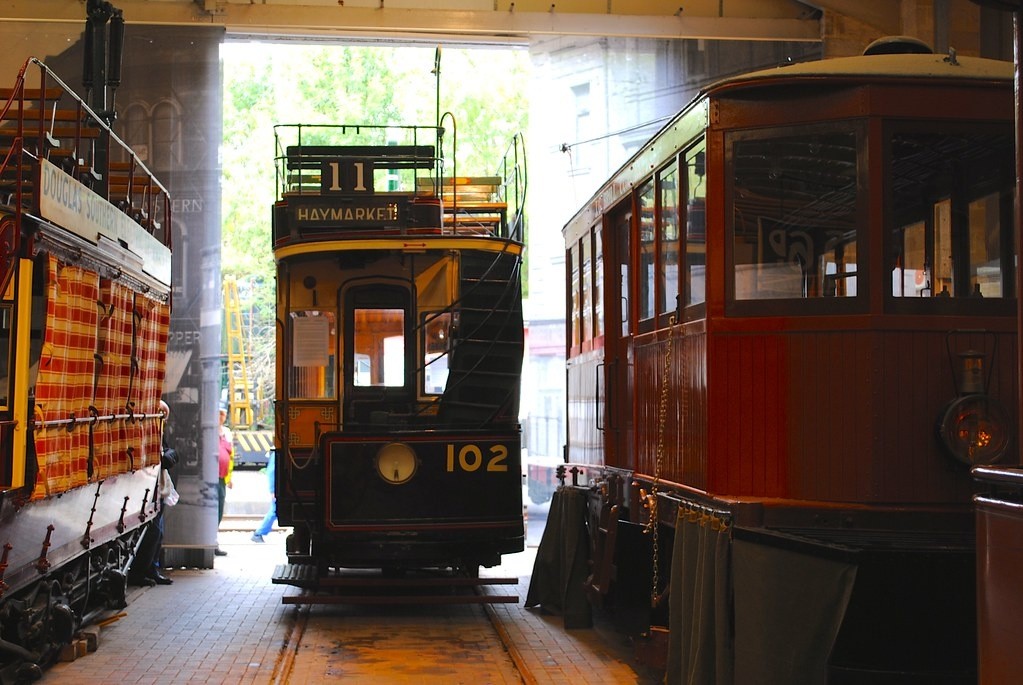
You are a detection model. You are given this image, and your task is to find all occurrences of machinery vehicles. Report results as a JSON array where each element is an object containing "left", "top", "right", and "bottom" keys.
[{"left": 220, "top": 274, "right": 278, "bottom": 467}]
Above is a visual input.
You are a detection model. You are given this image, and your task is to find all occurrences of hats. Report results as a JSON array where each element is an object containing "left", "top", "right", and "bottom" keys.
[{"left": 219, "top": 401, "right": 228, "bottom": 412}]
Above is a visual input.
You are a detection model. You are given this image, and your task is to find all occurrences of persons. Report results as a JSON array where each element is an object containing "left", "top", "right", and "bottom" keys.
[
  {"left": 251, "top": 433, "right": 275, "bottom": 544},
  {"left": 213, "top": 398, "right": 236, "bottom": 556}
]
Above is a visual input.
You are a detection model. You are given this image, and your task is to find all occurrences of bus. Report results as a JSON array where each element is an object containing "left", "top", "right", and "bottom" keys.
[
  {"left": 524, "top": 327, "right": 579, "bottom": 510},
  {"left": 525, "top": 35, "right": 1021, "bottom": 685},
  {"left": 1, "top": 0, "right": 181, "bottom": 684},
  {"left": 266, "top": 41, "right": 529, "bottom": 608}
]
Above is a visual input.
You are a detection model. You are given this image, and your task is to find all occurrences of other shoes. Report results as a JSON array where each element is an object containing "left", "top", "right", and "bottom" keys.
[
  {"left": 214, "top": 549, "right": 227, "bottom": 557},
  {"left": 249, "top": 533, "right": 265, "bottom": 546},
  {"left": 140, "top": 573, "right": 172, "bottom": 586}
]
